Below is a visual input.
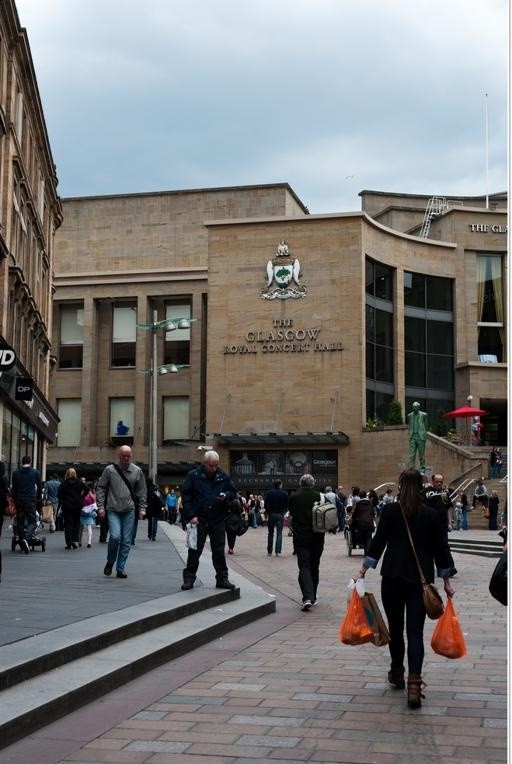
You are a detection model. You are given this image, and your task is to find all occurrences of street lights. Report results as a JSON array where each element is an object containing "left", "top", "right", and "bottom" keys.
[
  {"left": 137, "top": 359, "right": 192, "bottom": 478},
  {"left": 136, "top": 311, "right": 197, "bottom": 483}
]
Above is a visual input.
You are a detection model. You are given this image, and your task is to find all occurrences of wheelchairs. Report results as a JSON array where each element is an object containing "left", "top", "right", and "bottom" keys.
[{"left": 346, "top": 526, "right": 367, "bottom": 556}]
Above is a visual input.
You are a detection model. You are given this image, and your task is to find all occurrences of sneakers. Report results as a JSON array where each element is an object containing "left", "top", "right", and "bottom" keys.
[
  {"left": 180, "top": 578, "right": 195, "bottom": 590},
  {"left": 22, "top": 539, "right": 31, "bottom": 555},
  {"left": 103, "top": 563, "right": 113, "bottom": 575},
  {"left": 215, "top": 581, "right": 235, "bottom": 589},
  {"left": 117, "top": 570, "right": 128, "bottom": 579},
  {"left": 299, "top": 600, "right": 313, "bottom": 612}
]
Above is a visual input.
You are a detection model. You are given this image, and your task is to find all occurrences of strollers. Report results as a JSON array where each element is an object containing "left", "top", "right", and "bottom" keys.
[{"left": 11, "top": 509, "right": 46, "bottom": 551}]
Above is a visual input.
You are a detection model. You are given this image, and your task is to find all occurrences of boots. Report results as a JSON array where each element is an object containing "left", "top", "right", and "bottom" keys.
[
  {"left": 405, "top": 673, "right": 426, "bottom": 710},
  {"left": 385, "top": 666, "right": 408, "bottom": 690}
]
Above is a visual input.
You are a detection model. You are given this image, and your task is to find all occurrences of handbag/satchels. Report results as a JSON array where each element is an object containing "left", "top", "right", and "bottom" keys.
[
  {"left": 311, "top": 491, "right": 341, "bottom": 533},
  {"left": 5, "top": 489, "right": 17, "bottom": 517},
  {"left": 393, "top": 498, "right": 446, "bottom": 621}
]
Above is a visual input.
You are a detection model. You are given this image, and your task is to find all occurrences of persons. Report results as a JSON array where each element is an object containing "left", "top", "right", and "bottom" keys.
[
  {"left": 96, "top": 445, "right": 147, "bottom": 578},
  {"left": 0, "top": 461, "right": 10, "bottom": 538},
  {"left": 254, "top": 495, "right": 260, "bottom": 528},
  {"left": 12, "top": 455, "right": 41, "bottom": 553},
  {"left": 383, "top": 488, "right": 393, "bottom": 504},
  {"left": 264, "top": 479, "right": 288, "bottom": 556},
  {"left": 43, "top": 473, "right": 61, "bottom": 533},
  {"left": 226, "top": 496, "right": 240, "bottom": 553},
  {"left": 180, "top": 450, "right": 238, "bottom": 589},
  {"left": 57, "top": 467, "right": 90, "bottom": 550},
  {"left": 407, "top": 402, "right": 428, "bottom": 470},
  {"left": 324, "top": 486, "right": 337, "bottom": 534},
  {"left": 288, "top": 473, "right": 332, "bottom": 611},
  {"left": 79, "top": 489, "right": 96, "bottom": 548},
  {"left": 490, "top": 446, "right": 500, "bottom": 479},
  {"left": 353, "top": 492, "right": 375, "bottom": 559},
  {"left": 145, "top": 477, "right": 161, "bottom": 541},
  {"left": 352, "top": 486, "right": 360, "bottom": 511},
  {"left": 353, "top": 469, "right": 458, "bottom": 709},
  {"left": 489, "top": 490, "right": 499, "bottom": 530},
  {"left": 165, "top": 489, "right": 178, "bottom": 525},
  {"left": 470, "top": 481, "right": 488, "bottom": 510},
  {"left": 337, "top": 486, "right": 347, "bottom": 530},
  {"left": 369, "top": 490, "right": 378, "bottom": 516},
  {"left": 248, "top": 495, "right": 255, "bottom": 515},
  {"left": 460, "top": 490, "right": 467, "bottom": 530},
  {"left": 239, "top": 491, "right": 248, "bottom": 530},
  {"left": 423, "top": 473, "right": 454, "bottom": 542}
]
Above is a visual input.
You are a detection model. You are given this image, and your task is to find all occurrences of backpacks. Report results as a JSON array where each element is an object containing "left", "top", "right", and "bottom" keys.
[{"left": 313, "top": 493, "right": 336, "bottom": 534}]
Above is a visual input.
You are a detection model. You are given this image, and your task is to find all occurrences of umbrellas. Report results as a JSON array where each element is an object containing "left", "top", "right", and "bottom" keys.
[{"left": 444, "top": 406, "right": 487, "bottom": 443}]
